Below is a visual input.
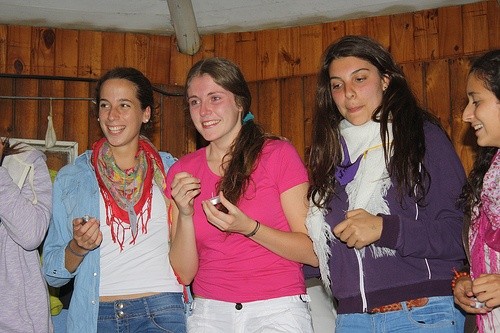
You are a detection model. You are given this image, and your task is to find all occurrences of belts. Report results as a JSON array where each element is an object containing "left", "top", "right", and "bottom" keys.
[{"left": 367, "top": 297, "right": 429, "bottom": 314}]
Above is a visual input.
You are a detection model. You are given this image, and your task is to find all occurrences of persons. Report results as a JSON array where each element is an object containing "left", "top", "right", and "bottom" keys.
[
  {"left": 164, "top": 58, "right": 320, "bottom": 333},
  {"left": 451, "top": 49, "right": 500, "bottom": 333},
  {"left": 0, "top": 137, "right": 55, "bottom": 333},
  {"left": 306, "top": 35, "right": 469, "bottom": 333},
  {"left": 41, "top": 66, "right": 188, "bottom": 333}
]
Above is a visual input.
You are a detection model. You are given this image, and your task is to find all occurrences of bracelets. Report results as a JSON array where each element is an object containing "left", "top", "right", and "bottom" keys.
[
  {"left": 246, "top": 220, "right": 260, "bottom": 237},
  {"left": 68, "top": 241, "right": 90, "bottom": 257},
  {"left": 452, "top": 271, "right": 468, "bottom": 291}
]
